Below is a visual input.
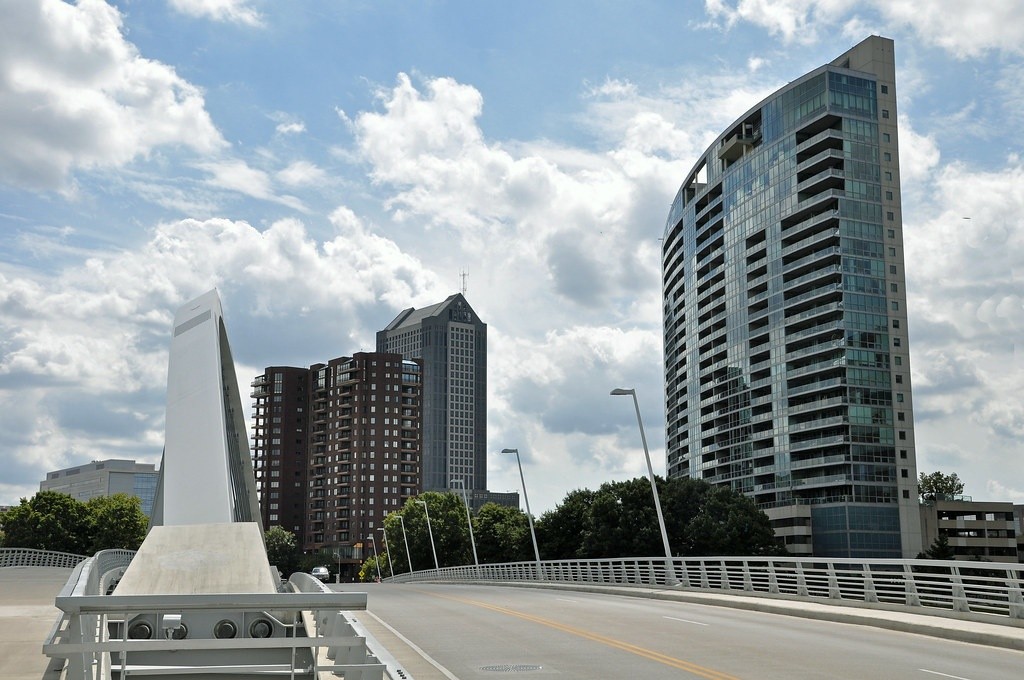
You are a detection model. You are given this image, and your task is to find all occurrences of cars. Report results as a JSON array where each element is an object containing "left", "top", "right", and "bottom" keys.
[{"left": 309, "top": 567, "right": 331, "bottom": 583}]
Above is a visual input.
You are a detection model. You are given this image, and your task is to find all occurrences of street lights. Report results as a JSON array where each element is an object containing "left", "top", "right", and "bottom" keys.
[
  {"left": 609, "top": 387, "right": 683, "bottom": 588},
  {"left": 415, "top": 500, "right": 441, "bottom": 581},
  {"left": 391, "top": 515, "right": 415, "bottom": 581},
  {"left": 502, "top": 449, "right": 546, "bottom": 584},
  {"left": 333, "top": 553, "right": 340, "bottom": 584},
  {"left": 366, "top": 537, "right": 382, "bottom": 584},
  {"left": 450, "top": 478, "right": 482, "bottom": 581},
  {"left": 376, "top": 528, "right": 394, "bottom": 582}
]
